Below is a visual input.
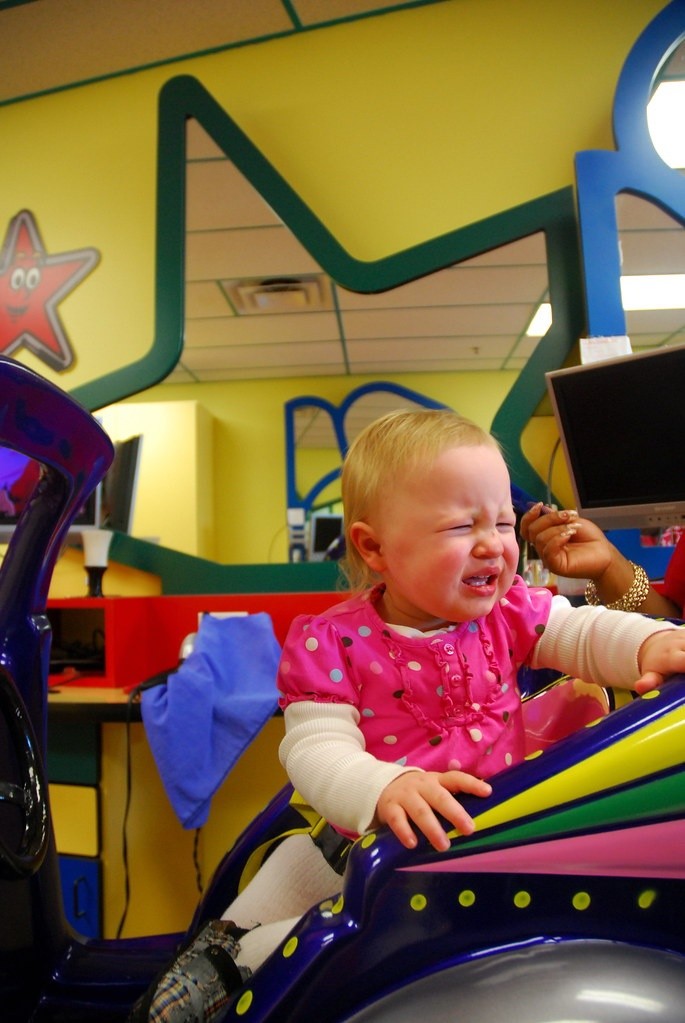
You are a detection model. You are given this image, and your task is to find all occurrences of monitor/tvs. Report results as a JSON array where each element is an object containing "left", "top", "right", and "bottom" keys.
[{"left": 545, "top": 344, "right": 685, "bottom": 531}]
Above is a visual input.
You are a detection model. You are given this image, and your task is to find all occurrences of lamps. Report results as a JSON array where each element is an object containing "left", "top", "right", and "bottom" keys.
[{"left": 82, "top": 530, "right": 113, "bottom": 597}]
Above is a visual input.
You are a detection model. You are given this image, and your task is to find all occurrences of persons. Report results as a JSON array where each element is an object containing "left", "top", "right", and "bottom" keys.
[
  {"left": 519, "top": 500, "right": 685, "bottom": 626},
  {"left": 147, "top": 408, "right": 685, "bottom": 1023}
]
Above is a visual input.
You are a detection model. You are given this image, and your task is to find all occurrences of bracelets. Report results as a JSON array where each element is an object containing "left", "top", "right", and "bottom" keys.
[{"left": 584, "top": 558, "right": 651, "bottom": 613}]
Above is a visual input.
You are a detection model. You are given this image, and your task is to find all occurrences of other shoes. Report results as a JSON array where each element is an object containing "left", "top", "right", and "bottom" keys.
[{"left": 134, "top": 920, "right": 262, "bottom": 1023}]
